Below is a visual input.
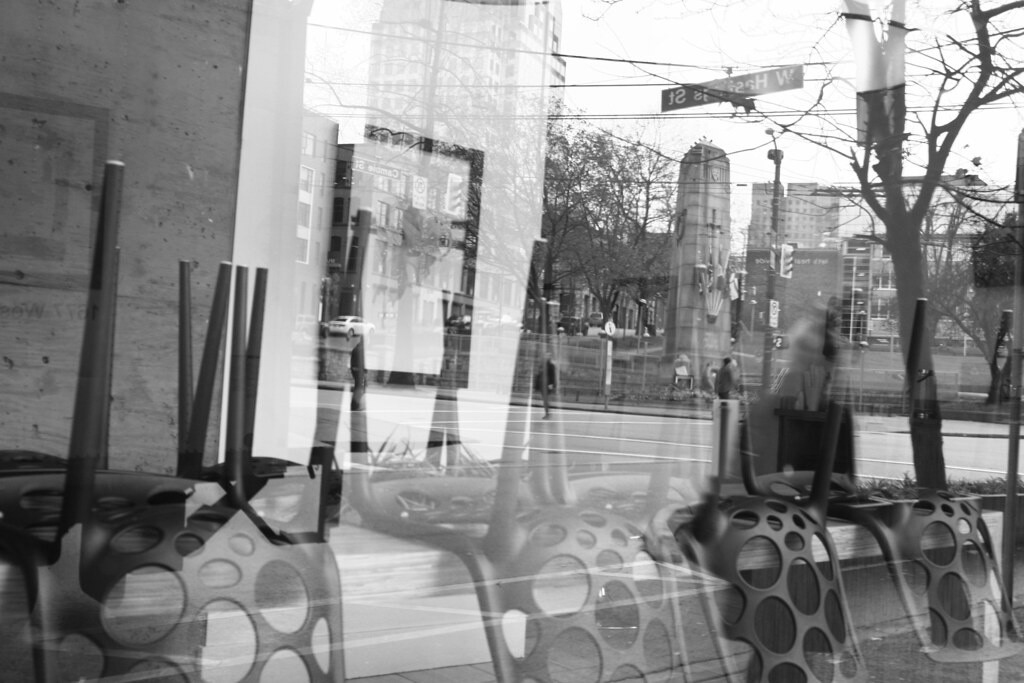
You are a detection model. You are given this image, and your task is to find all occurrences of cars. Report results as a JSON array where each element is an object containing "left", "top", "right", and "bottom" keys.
[
  {"left": 445, "top": 315, "right": 471, "bottom": 333},
  {"left": 325, "top": 312, "right": 376, "bottom": 337}
]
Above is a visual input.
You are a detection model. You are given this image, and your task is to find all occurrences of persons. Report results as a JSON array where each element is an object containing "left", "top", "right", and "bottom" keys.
[
  {"left": 532, "top": 352, "right": 558, "bottom": 419},
  {"left": 714, "top": 356, "right": 731, "bottom": 404},
  {"left": 347, "top": 337, "right": 369, "bottom": 411}
]
